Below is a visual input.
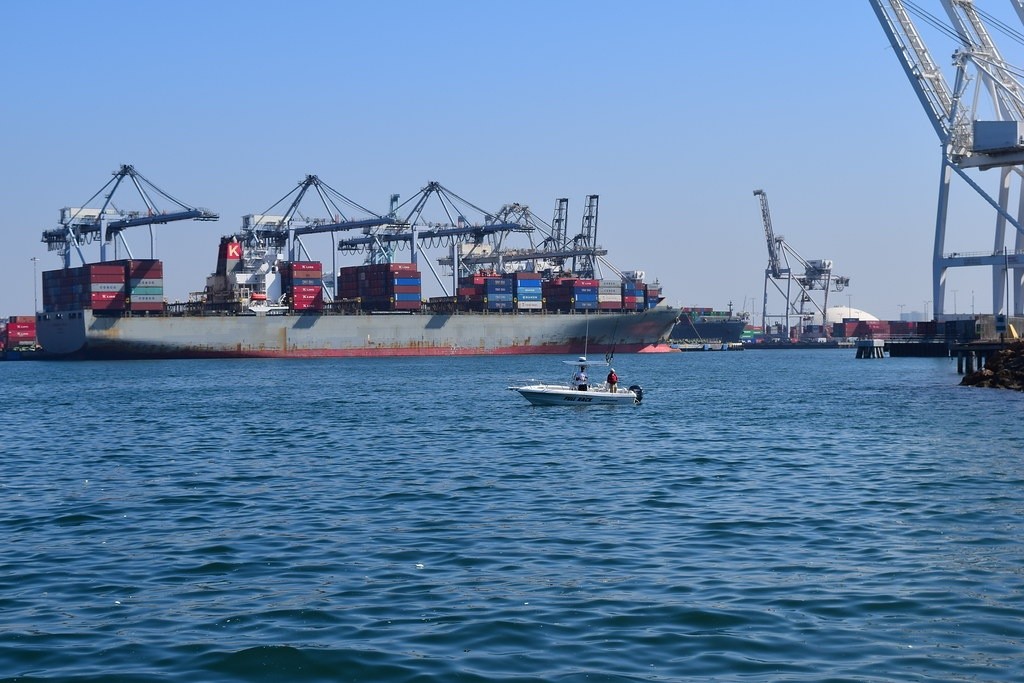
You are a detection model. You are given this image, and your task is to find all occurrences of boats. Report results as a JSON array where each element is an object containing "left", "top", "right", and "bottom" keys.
[{"left": 504, "top": 357, "right": 643, "bottom": 408}]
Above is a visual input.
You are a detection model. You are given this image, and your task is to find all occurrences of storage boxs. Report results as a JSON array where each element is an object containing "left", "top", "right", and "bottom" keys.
[
  {"left": 831, "top": 318, "right": 980, "bottom": 343},
  {"left": 450, "top": 269, "right": 666, "bottom": 312},
  {"left": 277, "top": 261, "right": 325, "bottom": 312},
  {"left": 41, "top": 258, "right": 166, "bottom": 314},
  {"left": 0, "top": 313, "right": 37, "bottom": 348},
  {"left": 336, "top": 262, "right": 424, "bottom": 311}
]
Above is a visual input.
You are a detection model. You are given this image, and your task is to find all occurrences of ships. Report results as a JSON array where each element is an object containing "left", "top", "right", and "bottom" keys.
[
  {"left": 33, "top": 162, "right": 686, "bottom": 356},
  {"left": 668, "top": 301, "right": 751, "bottom": 350}
]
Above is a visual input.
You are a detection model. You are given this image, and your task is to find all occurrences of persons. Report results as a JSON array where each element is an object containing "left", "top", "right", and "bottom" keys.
[
  {"left": 607, "top": 368, "right": 617, "bottom": 393},
  {"left": 576, "top": 366, "right": 587, "bottom": 392}
]
